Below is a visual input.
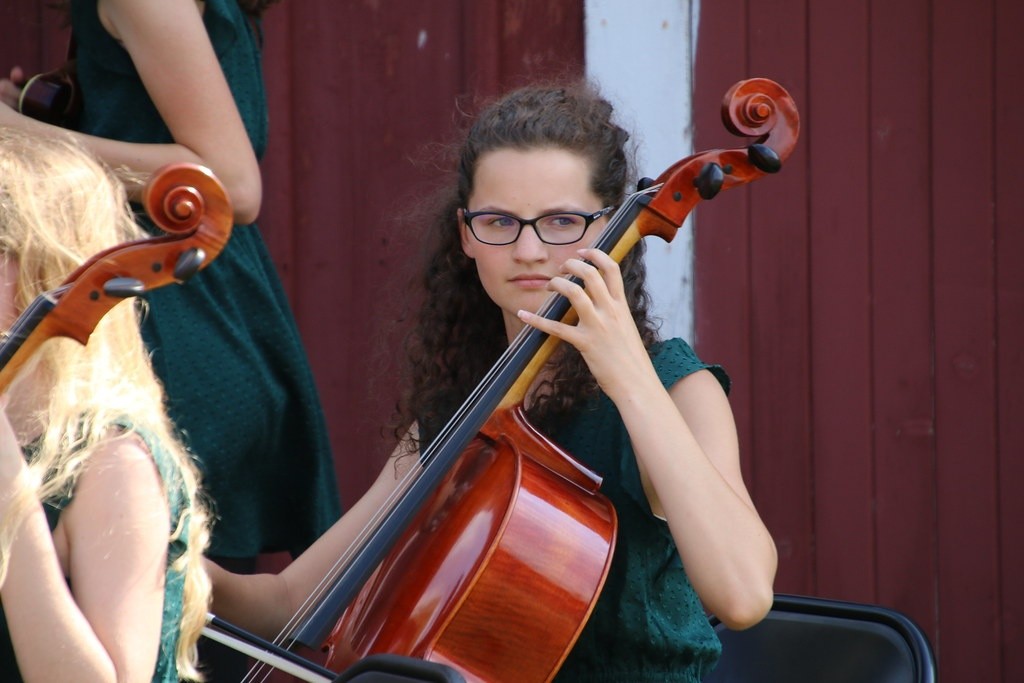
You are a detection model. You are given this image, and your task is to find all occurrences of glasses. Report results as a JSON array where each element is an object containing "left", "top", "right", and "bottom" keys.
[{"left": 463, "top": 206, "right": 615, "bottom": 245}]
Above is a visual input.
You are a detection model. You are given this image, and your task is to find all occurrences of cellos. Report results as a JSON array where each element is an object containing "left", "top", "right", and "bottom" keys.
[
  {"left": 0, "top": 161, "right": 235, "bottom": 401},
  {"left": 238, "top": 76, "right": 805, "bottom": 683}
]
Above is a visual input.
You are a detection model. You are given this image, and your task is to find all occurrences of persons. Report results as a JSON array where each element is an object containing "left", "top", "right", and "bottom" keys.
[
  {"left": 0, "top": 126, "right": 212, "bottom": 683},
  {"left": 201, "top": 80, "right": 779, "bottom": 683},
  {"left": 0, "top": 0, "right": 345, "bottom": 683}
]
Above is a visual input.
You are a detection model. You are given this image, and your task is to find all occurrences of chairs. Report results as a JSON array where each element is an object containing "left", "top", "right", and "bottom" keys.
[{"left": 702, "top": 593, "right": 937, "bottom": 683}]
